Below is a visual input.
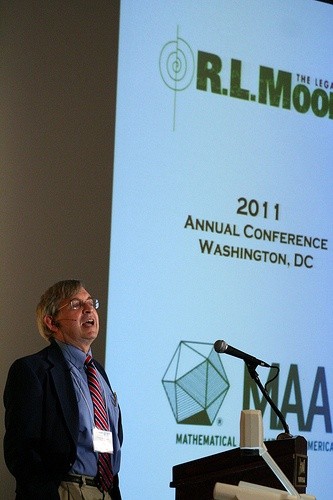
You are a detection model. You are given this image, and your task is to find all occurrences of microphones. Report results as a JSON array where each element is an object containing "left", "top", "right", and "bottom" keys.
[
  {"left": 214, "top": 340, "right": 272, "bottom": 367},
  {"left": 57, "top": 319, "right": 77, "bottom": 322}
]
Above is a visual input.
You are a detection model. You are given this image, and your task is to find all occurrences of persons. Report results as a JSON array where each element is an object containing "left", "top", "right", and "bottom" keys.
[{"left": 3, "top": 279, "right": 123, "bottom": 500}]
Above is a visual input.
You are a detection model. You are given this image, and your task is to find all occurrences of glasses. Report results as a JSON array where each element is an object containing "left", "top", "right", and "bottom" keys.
[{"left": 57, "top": 298, "right": 99, "bottom": 310}]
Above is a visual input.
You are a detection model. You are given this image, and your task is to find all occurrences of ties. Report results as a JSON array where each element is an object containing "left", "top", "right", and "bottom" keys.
[{"left": 84, "top": 353, "right": 113, "bottom": 492}]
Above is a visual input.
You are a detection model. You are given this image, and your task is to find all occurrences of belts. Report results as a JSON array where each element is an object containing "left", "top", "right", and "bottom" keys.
[{"left": 62, "top": 474, "right": 99, "bottom": 487}]
